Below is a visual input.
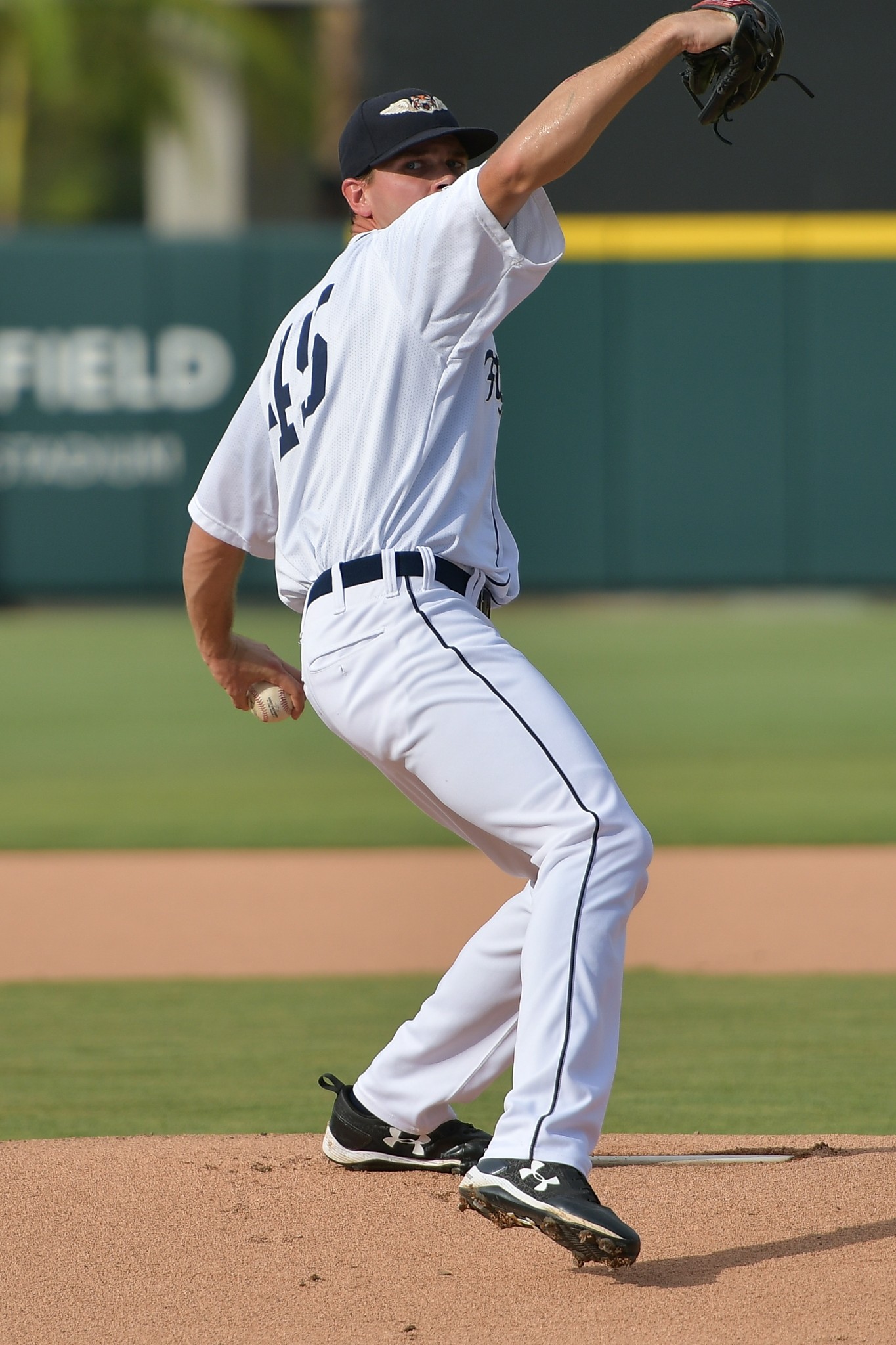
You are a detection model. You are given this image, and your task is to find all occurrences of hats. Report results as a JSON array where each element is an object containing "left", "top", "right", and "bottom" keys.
[{"left": 338, "top": 88, "right": 500, "bottom": 181}]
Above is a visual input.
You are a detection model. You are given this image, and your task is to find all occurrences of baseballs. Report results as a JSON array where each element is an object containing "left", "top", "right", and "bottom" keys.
[{"left": 246, "top": 680, "right": 293, "bottom": 724}]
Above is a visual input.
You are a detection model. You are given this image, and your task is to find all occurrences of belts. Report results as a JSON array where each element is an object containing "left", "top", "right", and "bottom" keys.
[{"left": 304, "top": 551, "right": 492, "bottom": 618}]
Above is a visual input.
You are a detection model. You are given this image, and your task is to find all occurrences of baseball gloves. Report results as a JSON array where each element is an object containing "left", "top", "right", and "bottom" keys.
[{"left": 680, "top": 0, "right": 786, "bottom": 124}]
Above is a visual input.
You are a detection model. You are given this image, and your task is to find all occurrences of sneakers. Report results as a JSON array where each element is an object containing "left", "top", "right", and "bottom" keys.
[
  {"left": 457, "top": 1156, "right": 641, "bottom": 1268},
  {"left": 317, "top": 1072, "right": 493, "bottom": 1176}
]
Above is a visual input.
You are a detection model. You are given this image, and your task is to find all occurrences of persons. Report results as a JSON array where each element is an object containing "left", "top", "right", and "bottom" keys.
[{"left": 182, "top": 0, "right": 784, "bottom": 1271}]
